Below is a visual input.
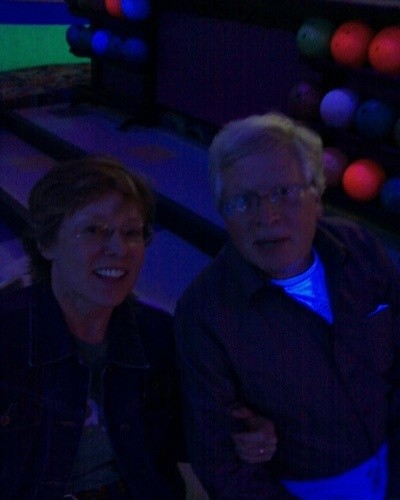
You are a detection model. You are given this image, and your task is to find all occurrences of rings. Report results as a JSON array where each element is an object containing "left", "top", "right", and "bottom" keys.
[{"left": 258, "top": 447, "right": 267, "bottom": 457}]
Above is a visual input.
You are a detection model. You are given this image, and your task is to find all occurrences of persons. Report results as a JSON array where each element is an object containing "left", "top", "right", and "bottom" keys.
[
  {"left": 174, "top": 115, "right": 400, "bottom": 500},
  {"left": 0, "top": 157, "right": 277, "bottom": 500}
]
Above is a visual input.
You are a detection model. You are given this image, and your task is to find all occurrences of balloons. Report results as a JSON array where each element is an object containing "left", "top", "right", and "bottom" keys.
[
  {"left": 64, "top": 0, "right": 149, "bottom": 65},
  {"left": 283, "top": 20, "right": 400, "bottom": 215}
]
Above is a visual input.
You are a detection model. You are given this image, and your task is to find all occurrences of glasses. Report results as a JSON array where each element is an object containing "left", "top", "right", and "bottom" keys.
[{"left": 220, "top": 180, "right": 314, "bottom": 221}]
